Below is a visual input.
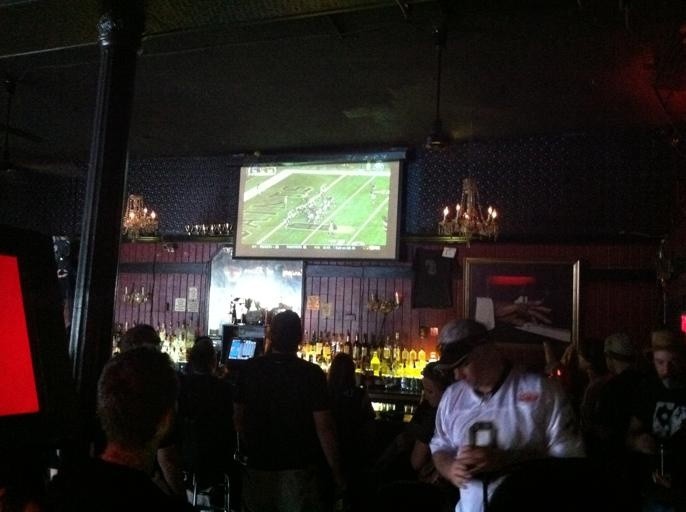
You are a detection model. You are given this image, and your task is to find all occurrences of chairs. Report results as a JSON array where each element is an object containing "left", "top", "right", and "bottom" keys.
[
  {"left": 486, "top": 456, "right": 686, "bottom": 512},
  {"left": 223, "top": 467, "right": 344, "bottom": 512}
]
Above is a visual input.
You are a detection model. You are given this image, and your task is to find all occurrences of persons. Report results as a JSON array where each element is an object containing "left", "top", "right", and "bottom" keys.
[{"left": 0, "top": 311, "right": 685, "bottom": 512}]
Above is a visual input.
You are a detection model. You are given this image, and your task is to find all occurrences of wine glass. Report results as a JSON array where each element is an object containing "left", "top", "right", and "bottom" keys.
[
  {"left": 193, "top": 225, "right": 202, "bottom": 235},
  {"left": 210, "top": 224, "right": 217, "bottom": 235},
  {"left": 201, "top": 224, "right": 210, "bottom": 235},
  {"left": 224, "top": 223, "right": 233, "bottom": 234},
  {"left": 218, "top": 224, "right": 225, "bottom": 235},
  {"left": 185, "top": 224, "right": 194, "bottom": 235}
]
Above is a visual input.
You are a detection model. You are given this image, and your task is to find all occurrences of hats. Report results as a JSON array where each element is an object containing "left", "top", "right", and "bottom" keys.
[
  {"left": 650, "top": 327, "right": 682, "bottom": 349},
  {"left": 272, "top": 311, "right": 303, "bottom": 335},
  {"left": 435, "top": 318, "right": 500, "bottom": 370},
  {"left": 601, "top": 334, "right": 638, "bottom": 360}
]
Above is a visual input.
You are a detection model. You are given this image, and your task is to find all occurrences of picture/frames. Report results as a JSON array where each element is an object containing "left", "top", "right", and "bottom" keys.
[{"left": 462, "top": 256, "right": 580, "bottom": 359}]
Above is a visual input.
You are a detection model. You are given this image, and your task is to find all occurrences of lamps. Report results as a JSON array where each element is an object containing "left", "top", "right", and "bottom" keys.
[
  {"left": 121, "top": 193, "right": 160, "bottom": 242},
  {"left": 425, "top": 25, "right": 449, "bottom": 150},
  {"left": 122, "top": 285, "right": 147, "bottom": 305},
  {"left": 438, "top": 176, "right": 499, "bottom": 249},
  {"left": 365, "top": 290, "right": 400, "bottom": 315}
]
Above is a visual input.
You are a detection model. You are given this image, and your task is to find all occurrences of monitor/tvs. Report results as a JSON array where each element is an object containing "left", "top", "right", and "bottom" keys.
[{"left": 225, "top": 336, "right": 265, "bottom": 364}]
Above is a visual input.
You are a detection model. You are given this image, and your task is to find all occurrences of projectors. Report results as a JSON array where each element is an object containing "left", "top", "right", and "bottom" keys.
[{"left": 424, "top": 134, "right": 450, "bottom": 154}]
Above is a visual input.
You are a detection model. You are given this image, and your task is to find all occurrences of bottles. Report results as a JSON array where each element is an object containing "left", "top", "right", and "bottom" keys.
[
  {"left": 296, "top": 329, "right": 440, "bottom": 422},
  {"left": 112, "top": 317, "right": 196, "bottom": 361}
]
